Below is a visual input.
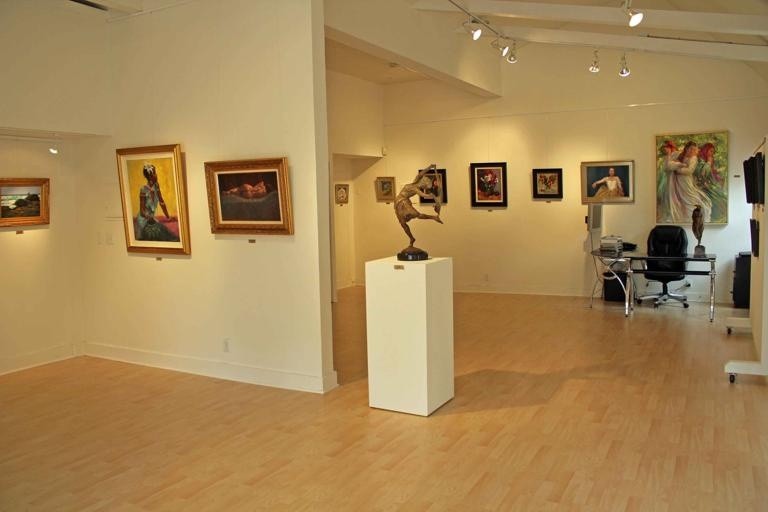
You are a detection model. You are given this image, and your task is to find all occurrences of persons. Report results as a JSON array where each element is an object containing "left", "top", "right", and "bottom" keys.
[
  {"left": 591, "top": 167, "right": 624, "bottom": 197},
  {"left": 394, "top": 165, "right": 443, "bottom": 248},
  {"left": 657, "top": 140, "right": 726, "bottom": 224},
  {"left": 222, "top": 181, "right": 273, "bottom": 199},
  {"left": 135, "top": 165, "right": 176, "bottom": 242}
]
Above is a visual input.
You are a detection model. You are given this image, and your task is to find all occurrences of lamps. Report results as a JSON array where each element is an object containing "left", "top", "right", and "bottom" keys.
[
  {"left": 585, "top": 50, "right": 602, "bottom": 76},
  {"left": 621, "top": 1, "right": 646, "bottom": 29},
  {"left": 617, "top": 54, "right": 632, "bottom": 80},
  {"left": 457, "top": 11, "right": 523, "bottom": 66}
]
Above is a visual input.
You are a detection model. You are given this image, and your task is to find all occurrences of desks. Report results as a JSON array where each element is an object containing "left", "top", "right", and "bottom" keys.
[{"left": 588, "top": 243, "right": 717, "bottom": 322}]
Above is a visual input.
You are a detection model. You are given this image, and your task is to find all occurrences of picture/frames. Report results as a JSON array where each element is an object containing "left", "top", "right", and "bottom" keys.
[
  {"left": 0, "top": 175, "right": 51, "bottom": 229},
  {"left": 531, "top": 167, "right": 565, "bottom": 202},
  {"left": 468, "top": 161, "right": 509, "bottom": 209},
  {"left": 335, "top": 183, "right": 349, "bottom": 205},
  {"left": 417, "top": 168, "right": 449, "bottom": 207},
  {"left": 204, "top": 156, "right": 297, "bottom": 237},
  {"left": 375, "top": 175, "right": 397, "bottom": 203},
  {"left": 652, "top": 127, "right": 732, "bottom": 229},
  {"left": 115, "top": 141, "right": 194, "bottom": 258},
  {"left": 578, "top": 158, "right": 637, "bottom": 207}
]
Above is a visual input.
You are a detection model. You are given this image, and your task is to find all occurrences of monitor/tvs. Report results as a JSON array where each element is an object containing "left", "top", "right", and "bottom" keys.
[{"left": 585, "top": 202, "right": 602, "bottom": 233}]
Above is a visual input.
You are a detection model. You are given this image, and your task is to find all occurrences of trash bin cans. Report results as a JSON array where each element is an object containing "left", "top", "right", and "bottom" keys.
[{"left": 603, "top": 270, "right": 628, "bottom": 302}]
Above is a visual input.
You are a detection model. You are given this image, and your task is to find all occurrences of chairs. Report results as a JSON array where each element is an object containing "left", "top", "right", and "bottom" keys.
[{"left": 636, "top": 221, "right": 692, "bottom": 308}]
